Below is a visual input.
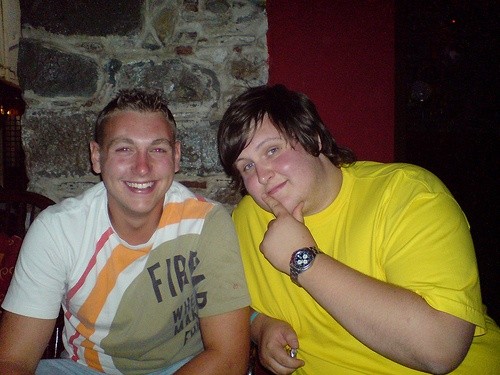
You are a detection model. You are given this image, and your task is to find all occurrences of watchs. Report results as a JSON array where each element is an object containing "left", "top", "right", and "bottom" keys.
[{"left": 288, "top": 246, "right": 325, "bottom": 287}]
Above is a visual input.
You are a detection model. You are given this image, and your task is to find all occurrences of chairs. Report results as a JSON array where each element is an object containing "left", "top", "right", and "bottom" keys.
[{"left": 0, "top": 189, "right": 65, "bottom": 359}]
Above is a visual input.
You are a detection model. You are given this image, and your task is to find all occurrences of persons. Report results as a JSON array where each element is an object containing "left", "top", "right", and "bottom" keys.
[
  {"left": 0, "top": 89, "right": 251, "bottom": 375},
  {"left": 217, "top": 83, "right": 500, "bottom": 375}
]
